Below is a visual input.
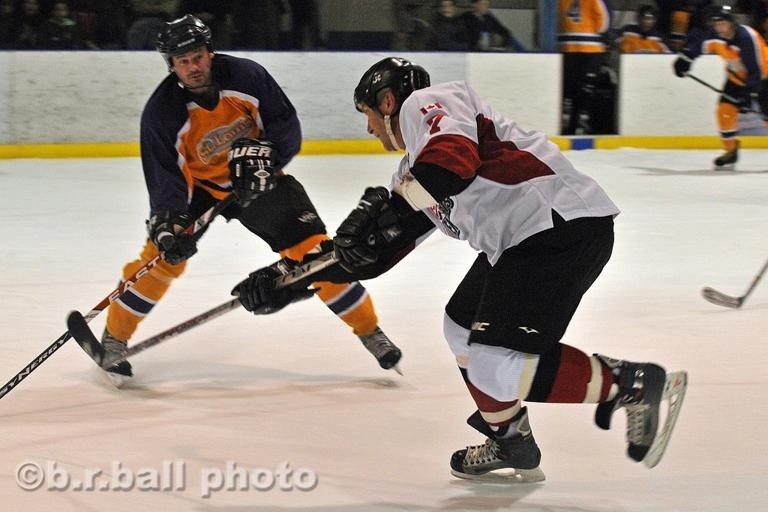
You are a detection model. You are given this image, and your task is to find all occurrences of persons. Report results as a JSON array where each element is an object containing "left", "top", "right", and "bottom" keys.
[
  {"left": 100, "top": 12, "right": 404, "bottom": 389},
  {"left": 230, "top": 56, "right": 688, "bottom": 485},
  {"left": 2, "top": 2, "right": 768, "bottom": 172}
]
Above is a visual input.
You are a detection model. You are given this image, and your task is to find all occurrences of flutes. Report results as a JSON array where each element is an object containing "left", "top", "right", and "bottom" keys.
[{"left": 67, "top": 250, "right": 344, "bottom": 371}]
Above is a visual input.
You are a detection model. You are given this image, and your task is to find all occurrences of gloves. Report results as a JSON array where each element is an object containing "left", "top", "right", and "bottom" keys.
[
  {"left": 673, "top": 49, "right": 694, "bottom": 77},
  {"left": 334, "top": 182, "right": 390, "bottom": 271},
  {"left": 224, "top": 136, "right": 280, "bottom": 208},
  {"left": 223, "top": 254, "right": 322, "bottom": 314},
  {"left": 142, "top": 208, "right": 199, "bottom": 265},
  {"left": 735, "top": 86, "right": 759, "bottom": 113}
]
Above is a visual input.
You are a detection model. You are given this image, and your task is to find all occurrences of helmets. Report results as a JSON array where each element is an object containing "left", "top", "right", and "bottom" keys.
[
  {"left": 155, "top": 13, "right": 214, "bottom": 72},
  {"left": 707, "top": 4, "right": 734, "bottom": 21},
  {"left": 352, "top": 55, "right": 431, "bottom": 121}
]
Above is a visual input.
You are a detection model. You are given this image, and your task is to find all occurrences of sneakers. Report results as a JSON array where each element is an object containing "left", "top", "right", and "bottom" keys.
[
  {"left": 358, "top": 326, "right": 402, "bottom": 370},
  {"left": 101, "top": 329, "right": 133, "bottom": 376}
]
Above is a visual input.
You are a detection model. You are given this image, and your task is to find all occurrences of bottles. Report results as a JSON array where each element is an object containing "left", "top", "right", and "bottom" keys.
[{"left": 479, "top": 33, "right": 491, "bottom": 51}]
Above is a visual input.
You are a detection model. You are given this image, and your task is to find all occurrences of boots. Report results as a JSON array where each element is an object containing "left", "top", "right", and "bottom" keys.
[
  {"left": 449, "top": 407, "right": 542, "bottom": 475},
  {"left": 714, "top": 141, "right": 738, "bottom": 166},
  {"left": 591, "top": 349, "right": 666, "bottom": 463}
]
